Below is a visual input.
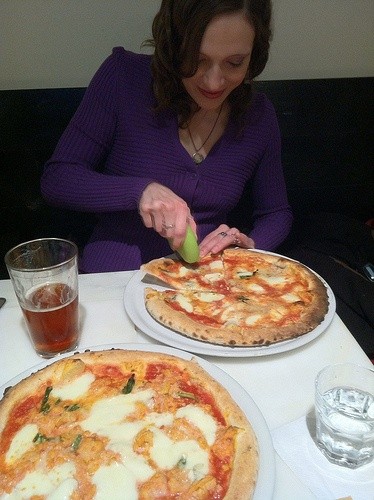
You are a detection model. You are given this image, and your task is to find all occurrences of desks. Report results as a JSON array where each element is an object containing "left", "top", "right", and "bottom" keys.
[{"left": 0, "top": 269, "right": 374, "bottom": 500}]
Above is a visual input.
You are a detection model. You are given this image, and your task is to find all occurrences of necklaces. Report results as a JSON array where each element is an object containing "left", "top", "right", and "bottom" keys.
[{"left": 184, "top": 100, "right": 224, "bottom": 163}]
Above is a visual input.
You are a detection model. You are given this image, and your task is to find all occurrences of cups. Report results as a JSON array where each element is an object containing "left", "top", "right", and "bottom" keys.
[
  {"left": 5, "top": 237, "right": 80, "bottom": 359},
  {"left": 316, "top": 363, "right": 374, "bottom": 468}
]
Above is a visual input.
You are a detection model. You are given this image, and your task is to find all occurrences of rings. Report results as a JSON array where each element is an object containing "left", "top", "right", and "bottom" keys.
[
  {"left": 164, "top": 224, "right": 174, "bottom": 229},
  {"left": 229, "top": 234, "right": 239, "bottom": 245},
  {"left": 218, "top": 232, "right": 228, "bottom": 237}
]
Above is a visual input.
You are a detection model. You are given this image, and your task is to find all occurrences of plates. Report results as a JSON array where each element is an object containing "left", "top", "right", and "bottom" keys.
[
  {"left": 123, "top": 247, "right": 335, "bottom": 358},
  {"left": 0, "top": 343, "right": 276, "bottom": 500}
]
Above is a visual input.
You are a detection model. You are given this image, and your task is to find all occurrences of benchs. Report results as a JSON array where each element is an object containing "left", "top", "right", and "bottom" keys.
[{"left": 0, "top": 76, "right": 373, "bottom": 363}]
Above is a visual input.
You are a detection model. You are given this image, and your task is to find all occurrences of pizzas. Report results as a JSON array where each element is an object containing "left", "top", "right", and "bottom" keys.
[
  {"left": 139, "top": 247, "right": 329, "bottom": 346},
  {"left": 0, "top": 349, "right": 260, "bottom": 500}
]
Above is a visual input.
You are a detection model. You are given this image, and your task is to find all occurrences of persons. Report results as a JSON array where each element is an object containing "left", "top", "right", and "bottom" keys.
[{"left": 40, "top": 0, "right": 294, "bottom": 274}]
